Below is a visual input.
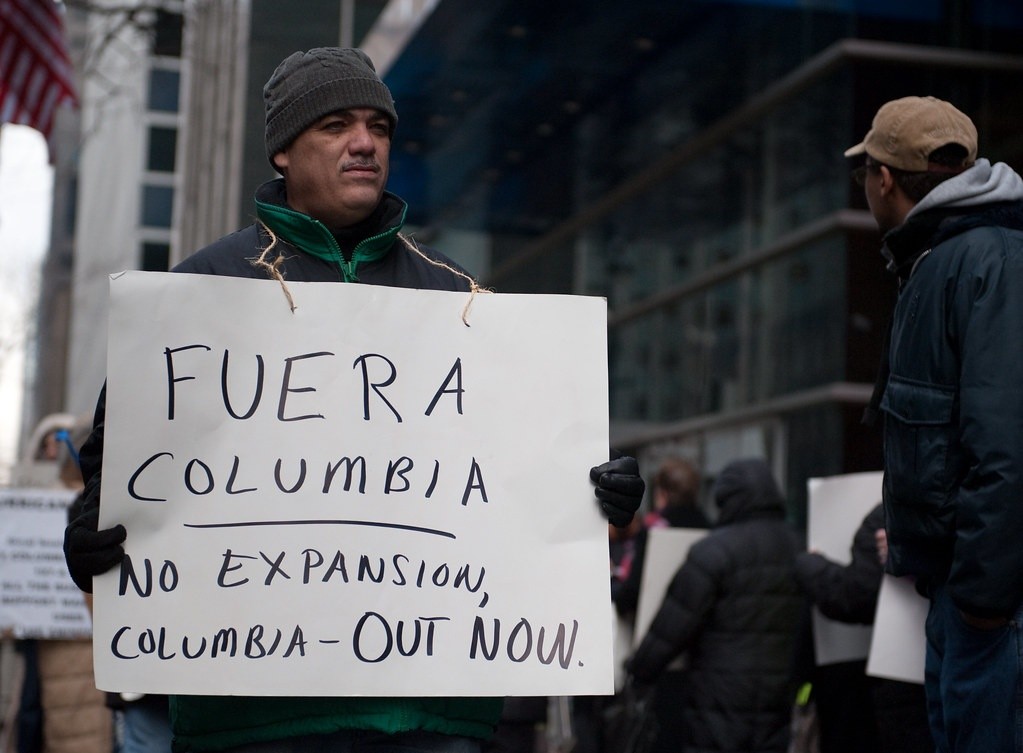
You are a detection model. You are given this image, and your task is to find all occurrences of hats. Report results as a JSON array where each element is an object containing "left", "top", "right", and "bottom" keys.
[
  {"left": 263, "top": 45, "right": 397, "bottom": 175},
  {"left": 843, "top": 93, "right": 979, "bottom": 178}
]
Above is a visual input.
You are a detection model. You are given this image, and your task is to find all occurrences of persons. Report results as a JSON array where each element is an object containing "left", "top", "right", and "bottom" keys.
[
  {"left": 62, "top": 48, "right": 647, "bottom": 753},
  {"left": 568, "top": 450, "right": 932, "bottom": 753},
  {"left": 844, "top": 96, "right": 1023, "bottom": 753},
  {"left": 104, "top": 693, "right": 173, "bottom": 753}
]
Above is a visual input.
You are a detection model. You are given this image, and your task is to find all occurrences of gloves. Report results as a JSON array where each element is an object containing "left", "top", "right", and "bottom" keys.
[
  {"left": 63, "top": 506, "right": 128, "bottom": 595},
  {"left": 589, "top": 456, "right": 646, "bottom": 532}
]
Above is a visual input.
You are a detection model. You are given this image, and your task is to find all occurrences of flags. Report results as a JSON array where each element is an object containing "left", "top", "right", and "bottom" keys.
[{"left": 0, "top": 0, "right": 77, "bottom": 141}]
